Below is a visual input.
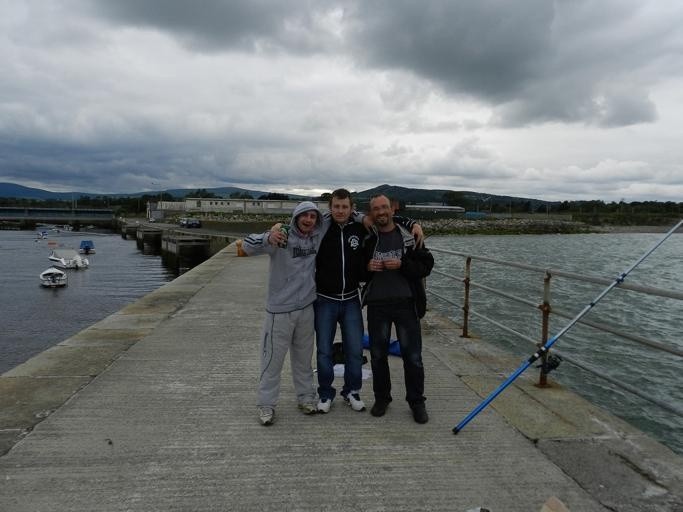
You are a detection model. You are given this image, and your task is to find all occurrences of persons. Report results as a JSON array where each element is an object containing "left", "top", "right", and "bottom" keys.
[
  {"left": 358, "top": 193, "right": 435, "bottom": 423},
  {"left": 242, "top": 201, "right": 373, "bottom": 426},
  {"left": 271, "top": 187, "right": 424, "bottom": 415}
]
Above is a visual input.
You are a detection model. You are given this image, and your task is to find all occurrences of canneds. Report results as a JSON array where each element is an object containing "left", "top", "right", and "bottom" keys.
[{"left": 278, "top": 224, "right": 290, "bottom": 249}]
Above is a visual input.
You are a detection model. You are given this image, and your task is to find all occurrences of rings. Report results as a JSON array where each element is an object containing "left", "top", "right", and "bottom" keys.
[
  {"left": 374, "top": 265, "right": 377, "bottom": 269},
  {"left": 377, "top": 262, "right": 379, "bottom": 265}
]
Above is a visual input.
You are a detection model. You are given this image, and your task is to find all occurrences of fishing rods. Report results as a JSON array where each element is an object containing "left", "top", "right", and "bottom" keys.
[{"left": 451, "top": 220, "right": 683, "bottom": 435}]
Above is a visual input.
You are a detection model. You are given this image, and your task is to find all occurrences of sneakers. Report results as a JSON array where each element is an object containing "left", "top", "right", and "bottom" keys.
[
  {"left": 317, "top": 398, "right": 331, "bottom": 413},
  {"left": 258, "top": 406, "right": 274, "bottom": 425},
  {"left": 344, "top": 390, "right": 366, "bottom": 411},
  {"left": 408, "top": 402, "right": 428, "bottom": 424},
  {"left": 298, "top": 401, "right": 316, "bottom": 414},
  {"left": 371, "top": 397, "right": 392, "bottom": 417}
]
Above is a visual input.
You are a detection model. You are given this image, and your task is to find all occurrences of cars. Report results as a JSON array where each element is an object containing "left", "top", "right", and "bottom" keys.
[
  {"left": 179, "top": 217, "right": 200, "bottom": 228},
  {"left": 150, "top": 217, "right": 155, "bottom": 223}
]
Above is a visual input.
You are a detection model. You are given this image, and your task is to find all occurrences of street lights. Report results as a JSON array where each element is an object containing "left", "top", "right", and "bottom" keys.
[{"left": 152, "top": 181, "right": 163, "bottom": 223}]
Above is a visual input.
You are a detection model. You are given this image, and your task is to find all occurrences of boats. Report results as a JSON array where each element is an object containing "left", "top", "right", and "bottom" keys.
[
  {"left": 79, "top": 240, "right": 97, "bottom": 255},
  {"left": 48, "top": 249, "right": 88, "bottom": 271},
  {"left": 35, "top": 223, "right": 94, "bottom": 239},
  {"left": 40, "top": 268, "right": 69, "bottom": 288}
]
